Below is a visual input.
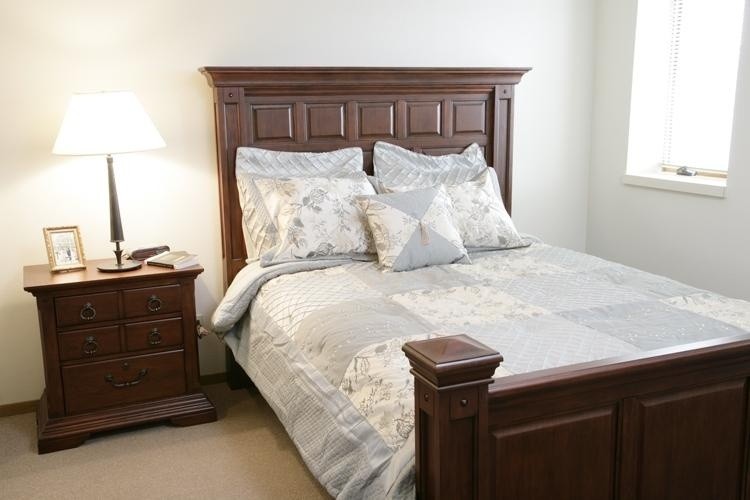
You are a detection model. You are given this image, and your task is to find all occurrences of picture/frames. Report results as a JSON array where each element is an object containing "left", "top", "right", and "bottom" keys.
[{"left": 43, "top": 224, "right": 86, "bottom": 274}]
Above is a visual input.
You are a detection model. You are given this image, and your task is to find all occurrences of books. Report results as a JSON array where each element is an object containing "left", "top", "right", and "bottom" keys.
[{"left": 145, "top": 249, "right": 201, "bottom": 269}]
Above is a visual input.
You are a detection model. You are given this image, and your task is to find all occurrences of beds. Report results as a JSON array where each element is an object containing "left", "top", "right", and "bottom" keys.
[{"left": 200, "top": 65, "right": 750, "bottom": 500}]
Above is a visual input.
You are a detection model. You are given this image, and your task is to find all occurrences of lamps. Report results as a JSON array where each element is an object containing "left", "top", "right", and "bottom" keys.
[{"left": 52, "top": 90, "right": 167, "bottom": 272}]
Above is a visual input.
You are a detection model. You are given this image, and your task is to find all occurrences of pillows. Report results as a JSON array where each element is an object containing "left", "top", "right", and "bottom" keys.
[{"left": 234, "top": 140, "right": 534, "bottom": 273}]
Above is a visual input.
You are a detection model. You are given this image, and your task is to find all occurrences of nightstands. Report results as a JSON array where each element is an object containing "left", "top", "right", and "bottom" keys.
[{"left": 23, "top": 255, "right": 218, "bottom": 455}]
